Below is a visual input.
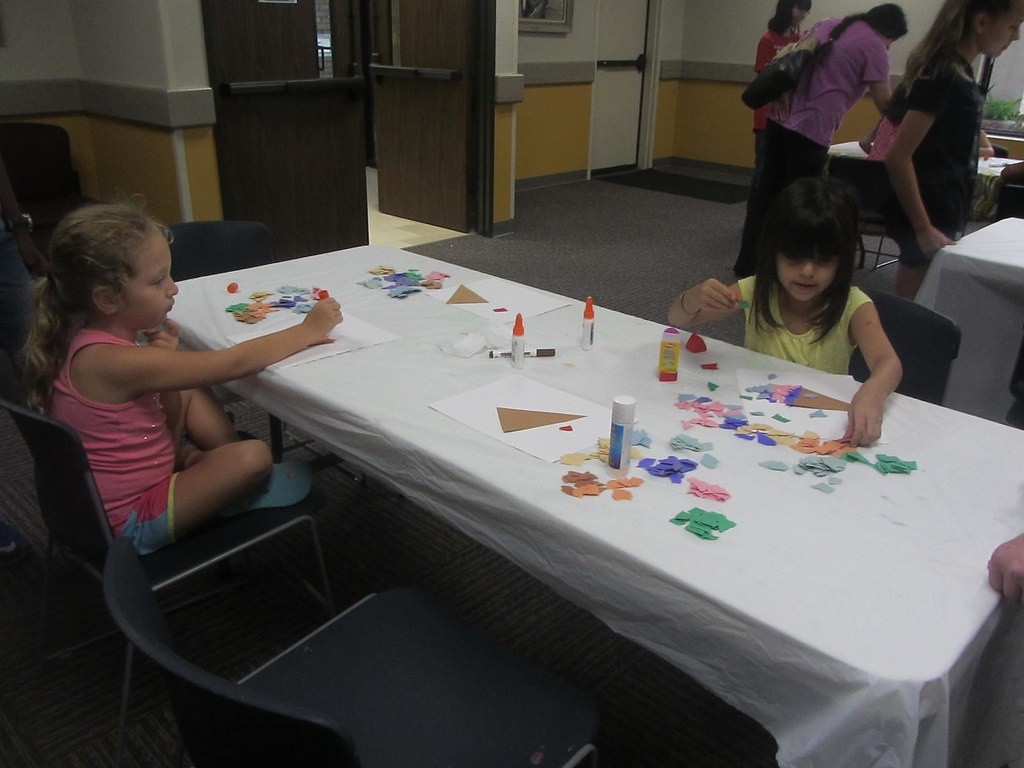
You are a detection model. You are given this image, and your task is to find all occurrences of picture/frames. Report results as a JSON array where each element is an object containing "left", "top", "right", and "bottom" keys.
[{"left": 518, "top": 0, "right": 574, "bottom": 34}]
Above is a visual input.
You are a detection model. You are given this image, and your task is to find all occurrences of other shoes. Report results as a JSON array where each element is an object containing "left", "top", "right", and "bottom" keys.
[
  {"left": 219, "top": 459, "right": 313, "bottom": 520},
  {"left": 176, "top": 410, "right": 234, "bottom": 464}
]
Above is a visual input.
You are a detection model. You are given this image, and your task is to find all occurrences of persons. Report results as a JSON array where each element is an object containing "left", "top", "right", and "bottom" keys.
[
  {"left": 734, "top": 3, "right": 909, "bottom": 277},
  {"left": 751, "top": 0, "right": 812, "bottom": 166},
  {"left": 976, "top": 129, "right": 994, "bottom": 157},
  {"left": 881, "top": 0, "right": 1024, "bottom": 300},
  {"left": 857, "top": 114, "right": 898, "bottom": 199},
  {"left": 667, "top": 179, "right": 902, "bottom": 449},
  {"left": 16, "top": 200, "right": 344, "bottom": 555}
]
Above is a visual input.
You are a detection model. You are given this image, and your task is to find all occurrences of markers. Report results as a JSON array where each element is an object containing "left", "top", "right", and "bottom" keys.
[{"left": 489, "top": 348, "right": 556, "bottom": 358}]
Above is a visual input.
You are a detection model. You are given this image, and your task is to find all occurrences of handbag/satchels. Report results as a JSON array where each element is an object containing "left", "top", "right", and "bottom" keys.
[{"left": 741, "top": 22, "right": 819, "bottom": 111}]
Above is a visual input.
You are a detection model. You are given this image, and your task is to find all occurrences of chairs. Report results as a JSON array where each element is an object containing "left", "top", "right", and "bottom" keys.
[
  {"left": 166, "top": 221, "right": 283, "bottom": 462},
  {"left": 831, "top": 156, "right": 900, "bottom": 269},
  {"left": 851, "top": 284, "right": 965, "bottom": 405},
  {"left": 993, "top": 185, "right": 1024, "bottom": 221},
  {"left": 0, "top": 120, "right": 98, "bottom": 229},
  {"left": 92, "top": 532, "right": 602, "bottom": 768},
  {"left": 0, "top": 398, "right": 333, "bottom": 768}
]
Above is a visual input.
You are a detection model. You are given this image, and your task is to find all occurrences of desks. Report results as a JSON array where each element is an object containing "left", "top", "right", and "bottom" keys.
[
  {"left": 161, "top": 243, "right": 1024, "bottom": 768},
  {"left": 907, "top": 215, "right": 1024, "bottom": 429},
  {"left": 828, "top": 139, "right": 1024, "bottom": 272}
]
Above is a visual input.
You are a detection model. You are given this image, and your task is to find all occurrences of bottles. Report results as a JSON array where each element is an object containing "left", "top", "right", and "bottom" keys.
[
  {"left": 511, "top": 312, "right": 525, "bottom": 369},
  {"left": 608, "top": 395, "right": 635, "bottom": 479},
  {"left": 582, "top": 296, "right": 594, "bottom": 350}
]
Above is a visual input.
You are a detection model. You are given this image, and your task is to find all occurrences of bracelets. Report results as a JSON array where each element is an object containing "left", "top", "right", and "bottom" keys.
[{"left": 681, "top": 292, "right": 702, "bottom": 315}]
